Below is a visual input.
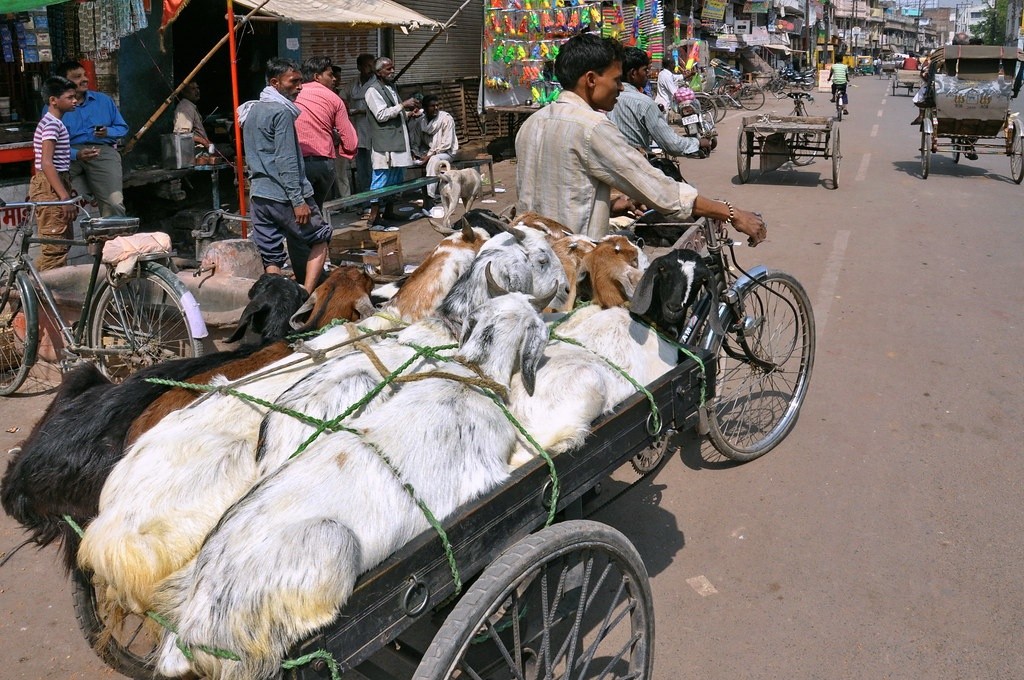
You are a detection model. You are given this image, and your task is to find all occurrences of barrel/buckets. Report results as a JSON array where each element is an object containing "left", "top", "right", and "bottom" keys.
[{"left": 161, "top": 128, "right": 195, "bottom": 169}]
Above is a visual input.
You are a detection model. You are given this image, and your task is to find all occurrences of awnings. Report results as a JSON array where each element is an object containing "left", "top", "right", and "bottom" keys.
[
  {"left": 123, "top": 0, "right": 469, "bottom": 239},
  {"left": 762, "top": 44, "right": 807, "bottom": 53}
]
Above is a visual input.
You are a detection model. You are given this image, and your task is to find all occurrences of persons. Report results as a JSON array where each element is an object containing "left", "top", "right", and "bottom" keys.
[
  {"left": 238, "top": 57, "right": 333, "bottom": 296},
  {"left": 173, "top": 77, "right": 236, "bottom": 162},
  {"left": 28, "top": 75, "right": 78, "bottom": 272},
  {"left": 902, "top": 52, "right": 918, "bottom": 92},
  {"left": 952, "top": 33, "right": 985, "bottom": 160},
  {"left": 873, "top": 56, "right": 882, "bottom": 74},
  {"left": 654, "top": 54, "right": 697, "bottom": 125},
  {"left": 332, "top": 54, "right": 460, "bottom": 228},
  {"left": 287, "top": 57, "right": 360, "bottom": 289},
  {"left": 827, "top": 55, "right": 850, "bottom": 114},
  {"left": 514, "top": 32, "right": 767, "bottom": 301},
  {"left": 41, "top": 60, "right": 129, "bottom": 217},
  {"left": 606, "top": 46, "right": 711, "bottom": 177}
]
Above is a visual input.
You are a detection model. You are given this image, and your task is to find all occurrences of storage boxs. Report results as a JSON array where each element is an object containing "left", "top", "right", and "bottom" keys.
[{"left": 332, "top": 231, "right": 403, "bottom": 275}]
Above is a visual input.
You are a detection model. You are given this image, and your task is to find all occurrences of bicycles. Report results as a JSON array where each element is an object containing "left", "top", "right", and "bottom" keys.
[
  {"left": 0, "top": 192, "right": 209, "bottom": 398},
  {"left": 643, "top": 139, "right": 714, "bottom": 183},
  {"left": 826, "top": 79, "right": 850, "bottom": 122},
  {"left": 673, "top": 60, "right": 819, "bottom": 139}
]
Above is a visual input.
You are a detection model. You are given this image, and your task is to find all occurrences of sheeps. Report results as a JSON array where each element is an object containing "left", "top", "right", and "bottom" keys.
[{"left": 0, "top": 212, "right": 728, "bottom": 680}]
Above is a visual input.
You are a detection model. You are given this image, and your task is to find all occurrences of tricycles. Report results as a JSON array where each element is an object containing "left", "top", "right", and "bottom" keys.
[
  {"left": 65, "top": 206, "right": 817, "bottom": 680},
  {"left": 736, "top": 91, "right": 843, "bottom": 190},
  {"left": 891, "top": 66, "right": 922, "bottom": 96},
  {"left": 909, "top": 42, "right": 1024, "bottom": 184}
]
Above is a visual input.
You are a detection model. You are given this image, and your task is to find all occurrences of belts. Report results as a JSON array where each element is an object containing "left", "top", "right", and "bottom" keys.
[
  {"left": 303, "top": 155, "right": 328, "bottom": 162},
  {"left": 84, "top": 142, "right": 126, "bottom": 149}
]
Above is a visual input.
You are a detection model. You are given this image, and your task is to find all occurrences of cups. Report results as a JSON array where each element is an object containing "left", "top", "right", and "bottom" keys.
[{"left": 207, "top": 144, "right": 215, "bottom": 154}]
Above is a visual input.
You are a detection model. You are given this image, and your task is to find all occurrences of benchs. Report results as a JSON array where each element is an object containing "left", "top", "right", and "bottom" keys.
[
  {"left": 325, "top": 176, "right": 440, "bottom": 226},
  {"left": 453, "top": 160, "right": 494, "bottom": 197}
]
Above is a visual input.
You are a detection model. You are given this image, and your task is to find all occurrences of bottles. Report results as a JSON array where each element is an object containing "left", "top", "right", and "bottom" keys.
[{"left": 11, "top": 109, "right": 18, "bottom": 121}]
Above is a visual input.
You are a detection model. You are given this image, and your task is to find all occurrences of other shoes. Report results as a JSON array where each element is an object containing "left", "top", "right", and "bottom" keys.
[
  {"left": 367, "top": 216, "right": 391, "bottom": 227},
  {"left": 830, "top": 98, "right": 836, "bottom": 102},
  {"left": 843, "top": 109, "right": 848, "bottom": 115},
  {"left": 910, "top": 116, "right": 923, "bottom": 125},
  {"left": 381, "top": 213, "right": 408, "bottom": 220},
  {"left": 965, "top": 146, "right": 978, "bottom": 160}
]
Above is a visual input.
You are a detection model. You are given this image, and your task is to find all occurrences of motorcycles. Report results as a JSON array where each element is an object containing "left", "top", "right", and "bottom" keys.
[{"left": 856, "top": 56, "right": 874, "bottom": 77}]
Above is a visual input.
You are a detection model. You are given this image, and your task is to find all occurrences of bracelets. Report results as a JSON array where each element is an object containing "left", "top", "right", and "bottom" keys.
[{"left": 726, "top": 200, "right": 733, "bottom": 226}]
